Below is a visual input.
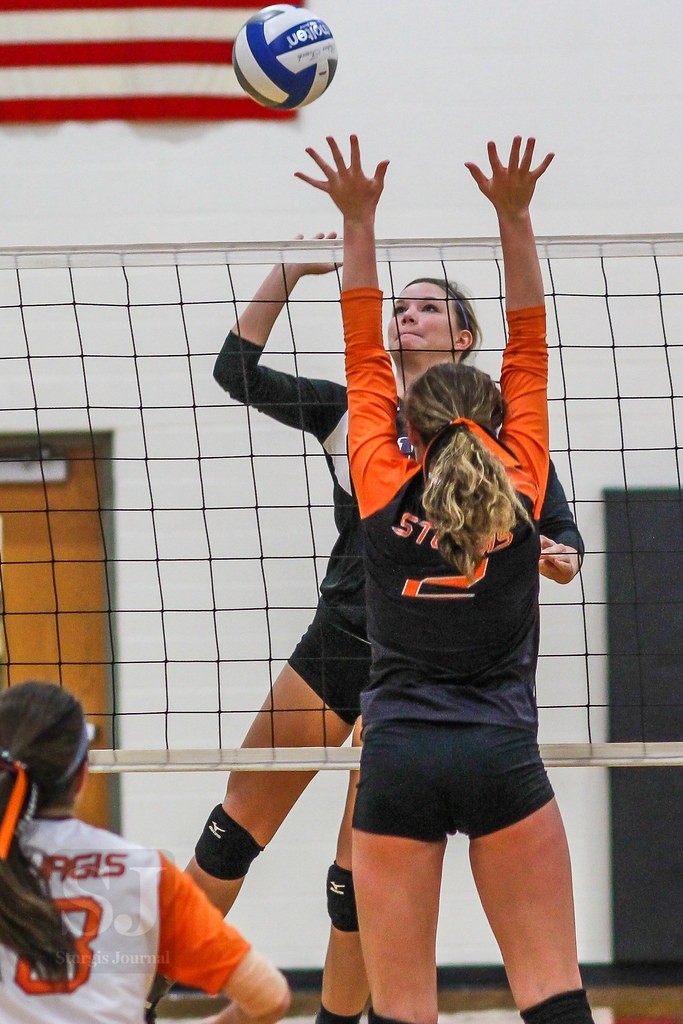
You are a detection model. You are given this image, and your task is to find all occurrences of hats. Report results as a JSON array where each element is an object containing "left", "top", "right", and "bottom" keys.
[{"left": 56, "top": 716, "right": 97, "bottom": 785}]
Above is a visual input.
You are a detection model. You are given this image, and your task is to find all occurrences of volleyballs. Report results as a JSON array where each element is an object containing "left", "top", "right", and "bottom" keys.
[{"left": 228, "top": 4, "right": 340, "bottom": 113}]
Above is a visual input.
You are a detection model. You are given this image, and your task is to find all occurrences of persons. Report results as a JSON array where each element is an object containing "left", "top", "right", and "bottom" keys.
[
  {"left": 0, "top": 672, "right": 291, "bottom": 1024},
  {"left": 295, "top": 134, "right": 594, "bottom": 1024},
  {"left": 146, "top": 226, "right": 585, "bottom": 1023}
]
